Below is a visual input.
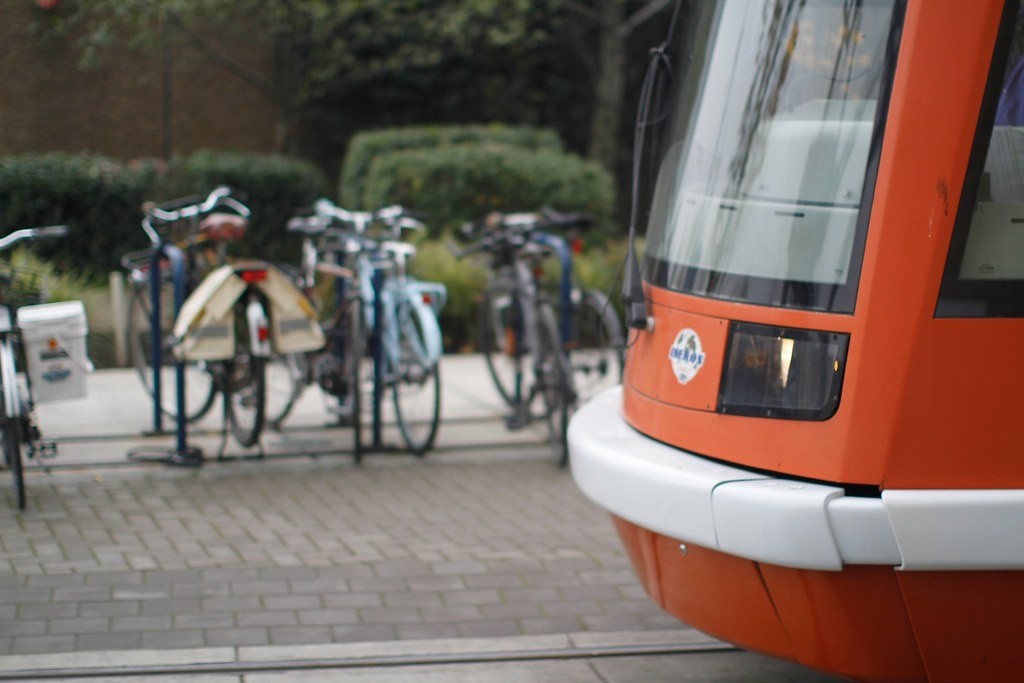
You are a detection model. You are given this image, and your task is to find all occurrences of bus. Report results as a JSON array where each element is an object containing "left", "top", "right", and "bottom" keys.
[{"left": 566, "top": 2, "right": 1024, "bottom": 683}]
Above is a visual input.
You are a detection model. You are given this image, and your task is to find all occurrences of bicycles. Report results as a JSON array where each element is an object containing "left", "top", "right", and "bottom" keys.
[
  {"left": 122, "top": 186, "right": 280, "bottom": 462},
  {"left": 284, "top": 198, "right": 450, "bottom": 456},
  {"left": 0, "top": 225, "right": 67, "bottom": 511},
  {"left": 447, "top": 204, "right": 627, "bottom": 469}
]
